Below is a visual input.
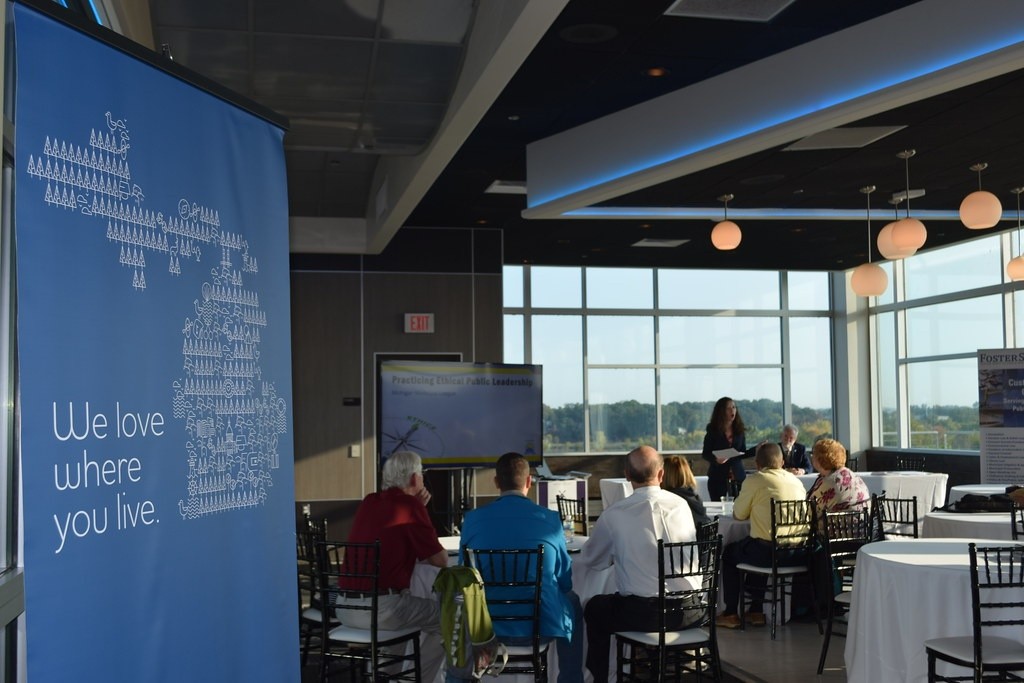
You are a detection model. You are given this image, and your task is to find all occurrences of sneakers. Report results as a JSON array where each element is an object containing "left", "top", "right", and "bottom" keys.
[
  {"left": 715, "top": 614, "right": 740, "bottom": 628},
  {"left": 744, "top": 611, "right": 766, "bottom": 626}
]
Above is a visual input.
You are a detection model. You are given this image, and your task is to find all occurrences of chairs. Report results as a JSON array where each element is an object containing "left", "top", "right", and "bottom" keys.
[{"left": 298, "top": 450, "right": 1024, "bottom": 683}]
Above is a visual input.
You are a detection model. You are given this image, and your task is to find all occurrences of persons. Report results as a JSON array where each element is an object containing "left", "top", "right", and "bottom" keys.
[
  {"left": 581, "top": 445, "right": 711, "bottom": 683},
  {"left": 777, "top": 424, "right": 812, "bottom": 475},
  {"left": 795, "top": 439, "right": 875, "bottom": 623},
  {"left": 660, "top": 454, "right": 715, "bottom": 596},
  {"left": 447, "top": 453, "right": 584, "bottom": 683},
  {"left": 702, "top": 397, "right": 768, "bottom": 502},
  {"left": 335, "top": 451, "right": 449, "bottom": 683},
  {"left": 714, "top": 442, "right": 809, "bottom": 629}
]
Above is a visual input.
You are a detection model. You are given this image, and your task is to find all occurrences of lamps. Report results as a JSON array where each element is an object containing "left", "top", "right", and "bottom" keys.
[
  {"left": 877, "top": 150, "right": 927, "bottom": 260},
  {"left": 851, "top": 185, "right": 888, "bottom": 295},
  {"left": 1008, "top": 187, "right": 1024, "bottom": 280},
  {"left": 960, "top": 162, "right": 1003, "bottom": 230},
  {"left": 711, "top": 194, "right": 742, "bottom": 250}
]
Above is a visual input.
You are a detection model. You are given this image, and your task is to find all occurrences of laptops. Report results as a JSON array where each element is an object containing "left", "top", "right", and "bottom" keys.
[{"left": 535, "top": 456, "right": 574, "bottom": 481}]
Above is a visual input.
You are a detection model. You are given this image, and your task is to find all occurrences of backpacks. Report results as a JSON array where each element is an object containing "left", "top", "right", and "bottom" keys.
[{"left": 432, "top": 549, "right": 508, "bottom": 680}]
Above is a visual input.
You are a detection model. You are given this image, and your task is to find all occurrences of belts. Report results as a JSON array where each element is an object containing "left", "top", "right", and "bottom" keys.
[{"left": 338, "top": 588, "right": 400, "bottom": 599}]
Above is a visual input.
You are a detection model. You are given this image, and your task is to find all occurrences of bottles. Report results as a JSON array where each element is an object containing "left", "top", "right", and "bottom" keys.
[{"left": 563, "top": 515, "right": 575, "bottom": 543}]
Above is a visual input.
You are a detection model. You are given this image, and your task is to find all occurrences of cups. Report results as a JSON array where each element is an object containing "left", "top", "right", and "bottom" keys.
[{"left": 720, "top": 496, "right": 734, "bottom": 515}]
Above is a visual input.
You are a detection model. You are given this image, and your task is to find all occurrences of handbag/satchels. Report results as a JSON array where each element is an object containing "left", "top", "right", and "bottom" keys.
[{"left": 932, "top": 494, "right": 1014, "bottom": 513}]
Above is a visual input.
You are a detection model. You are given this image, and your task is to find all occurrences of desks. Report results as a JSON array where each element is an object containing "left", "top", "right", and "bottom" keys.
[
  {"left": 844, "top": 538, "right": 1024, "bottom": 683},
  {"left": 922, "top": 511, "right": 1024, "bottom": 540},
  {"left": 401, "top": 533, "right": 632, "bottom": 683},
  {"left": 599, "top": 476, "right": 713, "bottom": 512},
  {"left": 536, "top": 476, "right": 590, "bottom": 537},
  {"left": 949, "top": 484, "right": 1024, "bottom": 510},
  {"left": 703, "top": 501, "right": 793, "bottom": 626},
  {"left": 796, "top": 471, "right": 949, "bottom": 540}
]
